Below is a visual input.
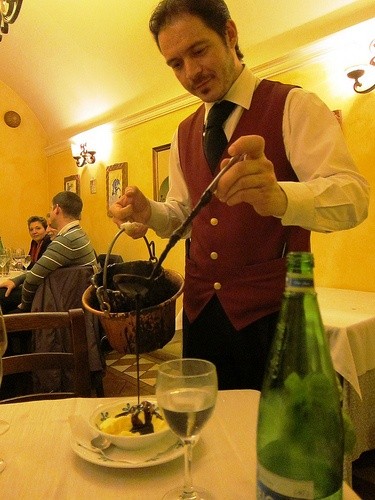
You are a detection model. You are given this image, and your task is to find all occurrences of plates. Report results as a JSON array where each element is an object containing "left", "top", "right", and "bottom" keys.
[{"left": 68, "top": 418, "right": 203, "bottom": 472}]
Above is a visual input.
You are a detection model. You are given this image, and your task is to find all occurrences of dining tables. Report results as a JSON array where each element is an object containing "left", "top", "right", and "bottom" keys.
[
  {"left": 0, "top": 261, "right": 29, "bottom": 290},
  {"left": 0, "top": 388, "right": 364, "bottom": 500}
]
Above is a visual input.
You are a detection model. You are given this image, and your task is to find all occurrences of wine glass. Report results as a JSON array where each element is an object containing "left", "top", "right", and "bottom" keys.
[
  {"left": 1, "top": 236, "right": 31, "bottom": 280},
  {"left": 154, "top": 358, "right": 217, "bottom": 500}
]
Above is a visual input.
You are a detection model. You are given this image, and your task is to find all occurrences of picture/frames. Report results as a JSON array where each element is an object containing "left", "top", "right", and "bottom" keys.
[
  {"left": 64, "top": 175, "right": 82, "bottom": 221},
  {"left": 152, "top": 143, "right": 172, "bottom": 203},
  {"left": 105, "top": 162, "right": 128, "bottom": 218}
]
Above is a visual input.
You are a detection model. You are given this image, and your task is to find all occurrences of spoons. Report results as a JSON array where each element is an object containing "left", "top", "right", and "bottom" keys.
[{"left": 90, "top": 435, "right": 110, "bottom": 451}]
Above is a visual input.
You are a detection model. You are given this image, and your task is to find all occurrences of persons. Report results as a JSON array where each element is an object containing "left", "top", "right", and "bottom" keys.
[
  {"left": 106, "top": 0, "right": 371, "bottom": 392},
  {"left": 20, "top": 216, "right": 54, "bottom": 272},
  {"left": 0, "top": 191, "right": 100, "bottom": 312}
]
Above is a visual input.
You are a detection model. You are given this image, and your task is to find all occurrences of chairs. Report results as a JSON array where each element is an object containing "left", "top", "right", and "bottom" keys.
[{"left": 0, "top": 308, "right": 92, "bottom": 403}]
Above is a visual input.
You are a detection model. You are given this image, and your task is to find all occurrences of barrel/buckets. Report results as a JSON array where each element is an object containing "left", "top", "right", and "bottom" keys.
[
  {"left": 90, "top": 260, "right": 165, "bottom": 313},
  {"left": 81, "top": 226, "right": 187, "bottom": 355}
]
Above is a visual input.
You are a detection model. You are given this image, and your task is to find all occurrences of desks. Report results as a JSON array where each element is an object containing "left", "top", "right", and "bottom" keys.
[{"left": 309, "top": 286, "right": 375, "bottom": 490}]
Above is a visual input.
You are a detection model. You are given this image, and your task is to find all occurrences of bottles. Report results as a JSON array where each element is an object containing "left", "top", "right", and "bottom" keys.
[{"left": 254, "top": 250, "right": 346, "bottom": 500}]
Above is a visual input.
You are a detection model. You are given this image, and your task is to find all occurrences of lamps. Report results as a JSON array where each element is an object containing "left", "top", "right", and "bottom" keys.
[
  {"left": 344, "top": 38, "right": 375, "bottom": 95},
  {"left": 0, "top": 0, "right": 23, "bottom": 41},
  {"left": 73, "top": 142, "right": 97, "bottom": 168}
]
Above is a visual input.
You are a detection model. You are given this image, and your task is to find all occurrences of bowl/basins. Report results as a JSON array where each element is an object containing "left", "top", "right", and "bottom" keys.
[{"left": 88, "top": 399, "right": 171, "bottom": 451}]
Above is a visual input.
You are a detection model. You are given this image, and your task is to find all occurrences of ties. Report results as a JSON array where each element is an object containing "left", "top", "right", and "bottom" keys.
[{"left": 203, "top": 100, "right": 236, "bottom": 175}]
